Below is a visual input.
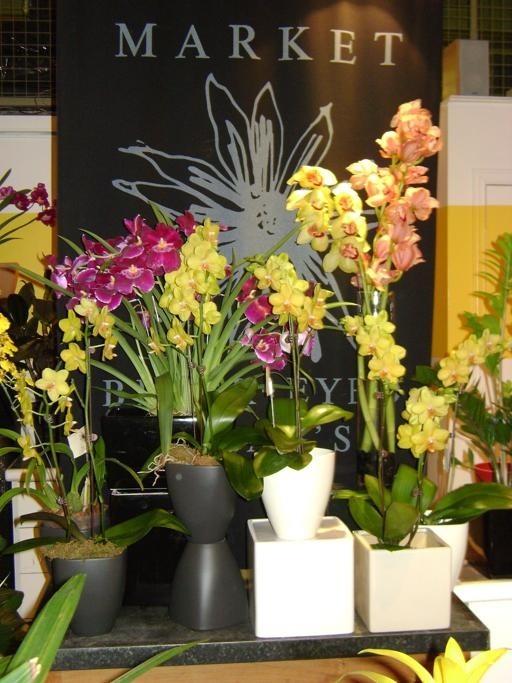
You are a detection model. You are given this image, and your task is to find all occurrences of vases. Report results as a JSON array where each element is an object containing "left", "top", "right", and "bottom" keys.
[
  {"left": 165, "top": 463, "right": 249, "bottom": 634},
  {"left": 353, "top": 526, "right": 455, "bottom": 634},
  {"left": 5, "top": 455, "right": 90, "bottom": 623},
  {"left": 46, "top": 554, "right": 127, "bottom": 638},
  {"left": 421, "top": 522, "right": 472, "bottom": 583},
  {"left": 482, "top": 484, "right": 512, "bottom": 580},
  {"left": 247, "top": 448, "right": 353, "bottom": 540}
]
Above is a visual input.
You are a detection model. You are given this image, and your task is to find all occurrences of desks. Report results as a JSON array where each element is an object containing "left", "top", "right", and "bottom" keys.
[{"left": 45, "top": 591, "right": 490, "bottom": 683}]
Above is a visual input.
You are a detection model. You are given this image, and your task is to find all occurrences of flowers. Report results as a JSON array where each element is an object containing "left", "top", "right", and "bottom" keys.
[
  {"left": 411, "top": 232, "right": 512, "bottom": 487},
  {"left": 239, "top": 395, "right": 357, "bottom": 476},
  {"left": 41, "top": 207, "right": 327, "bottom": 464},
  {"left": 285, "top": 99, "right": 512, "bottom": 551},
  {"left": 0, "top": 175, "right": 194, "bottom": 558}
]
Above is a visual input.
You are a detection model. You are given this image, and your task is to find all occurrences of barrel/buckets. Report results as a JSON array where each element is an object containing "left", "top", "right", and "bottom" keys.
[{"left": 474, "top": 462, "right": 512, "bottom": 484}]
